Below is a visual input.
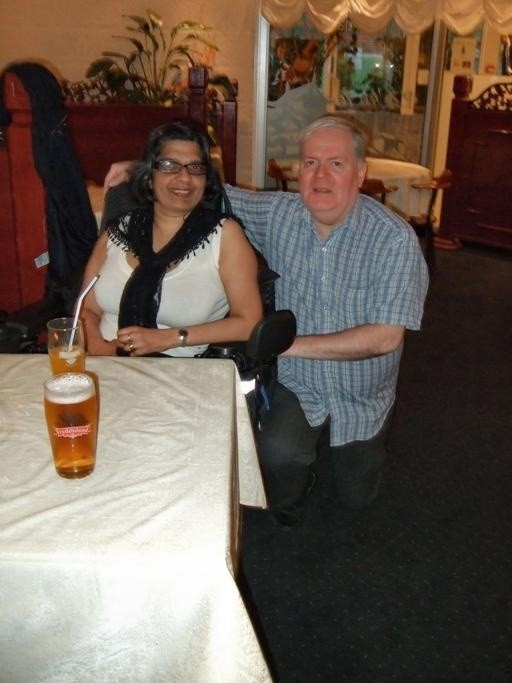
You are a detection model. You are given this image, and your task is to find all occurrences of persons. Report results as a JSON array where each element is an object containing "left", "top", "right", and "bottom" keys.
[
  {"left": 69, "top": 113, "right": 268, "bottom": 362},
  {"left": 103, "top": 110, "right": 431, "bottom": 530}
]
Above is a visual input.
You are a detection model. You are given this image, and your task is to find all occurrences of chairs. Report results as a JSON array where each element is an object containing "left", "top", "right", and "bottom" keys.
[{"left": 266, "top": 157, "right": 454, "bottom": 280}]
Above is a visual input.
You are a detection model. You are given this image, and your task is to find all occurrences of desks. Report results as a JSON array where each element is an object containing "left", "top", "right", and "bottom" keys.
[{"left": 1, "top": 353, "right": 235, "bottom": 581}]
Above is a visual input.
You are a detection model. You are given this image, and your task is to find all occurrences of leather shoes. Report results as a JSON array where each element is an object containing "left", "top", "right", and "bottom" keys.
[{"left": 274, "top": 472, "right": 317, "bottom": 526}]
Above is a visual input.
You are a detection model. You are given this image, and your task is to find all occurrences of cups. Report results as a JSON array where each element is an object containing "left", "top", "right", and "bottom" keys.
[
  {"left": 47, "top": 316, "right": 87, "bottom": 375},
  {"left": 43, "top": 371, "right": 101, "bottom": 480}
]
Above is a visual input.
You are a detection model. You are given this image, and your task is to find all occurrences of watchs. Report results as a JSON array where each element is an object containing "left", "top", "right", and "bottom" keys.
[{"left": 176, "top": 325, "right": 189, "bottom": 347}]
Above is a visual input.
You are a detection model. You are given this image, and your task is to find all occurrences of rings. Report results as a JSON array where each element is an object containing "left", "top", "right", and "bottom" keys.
[
  {"left": 129, "top": 342, "right": 135, "bottom": 351},
  {"left": 127, "top": 334, "right": 132, "bottom": 343}
]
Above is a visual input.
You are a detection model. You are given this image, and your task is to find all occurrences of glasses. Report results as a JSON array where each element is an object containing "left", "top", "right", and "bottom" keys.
[{"left": 152, "top": 159, "right": 211, "bottom": 176}]
